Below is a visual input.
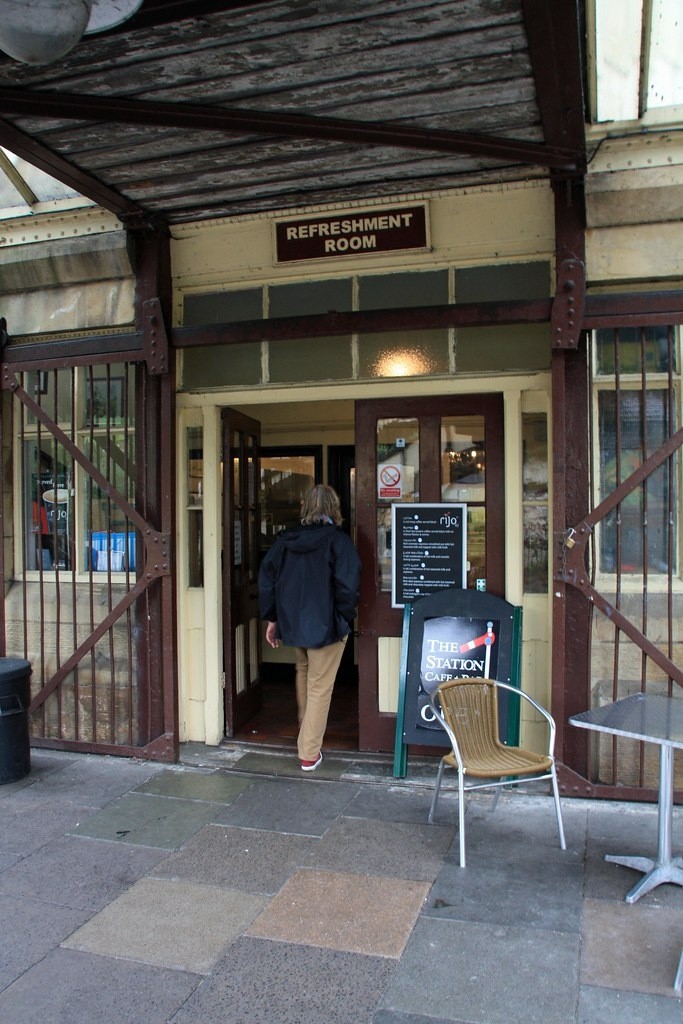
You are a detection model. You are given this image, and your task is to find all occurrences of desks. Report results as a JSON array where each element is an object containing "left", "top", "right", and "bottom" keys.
[{"left": 567, "top": 692, "right": 683, "bottom": 904}]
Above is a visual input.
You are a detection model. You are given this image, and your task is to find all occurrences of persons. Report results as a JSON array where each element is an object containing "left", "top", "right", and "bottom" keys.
[{"left": 262, "top": 483, "right": 365, "bottom": 771}]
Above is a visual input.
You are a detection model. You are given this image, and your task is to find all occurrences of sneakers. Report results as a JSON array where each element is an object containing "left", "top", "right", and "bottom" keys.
[{"left": 301, "top": 750, "right": 322, "bottom": 771}]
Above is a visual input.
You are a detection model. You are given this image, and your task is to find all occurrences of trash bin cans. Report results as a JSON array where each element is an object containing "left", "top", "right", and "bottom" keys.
[{"left": 0, "top": 656, "right": 34, "bottom": 787}]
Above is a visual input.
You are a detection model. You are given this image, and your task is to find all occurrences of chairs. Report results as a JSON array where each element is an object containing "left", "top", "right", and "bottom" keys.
[{"left": 428, "top": 678, "right": 566, "bottom": 868}]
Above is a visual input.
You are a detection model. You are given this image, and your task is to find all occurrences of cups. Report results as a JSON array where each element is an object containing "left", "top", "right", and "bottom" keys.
[{"left": 42, "top": 489, "right": 74, "bottom": 534}]
